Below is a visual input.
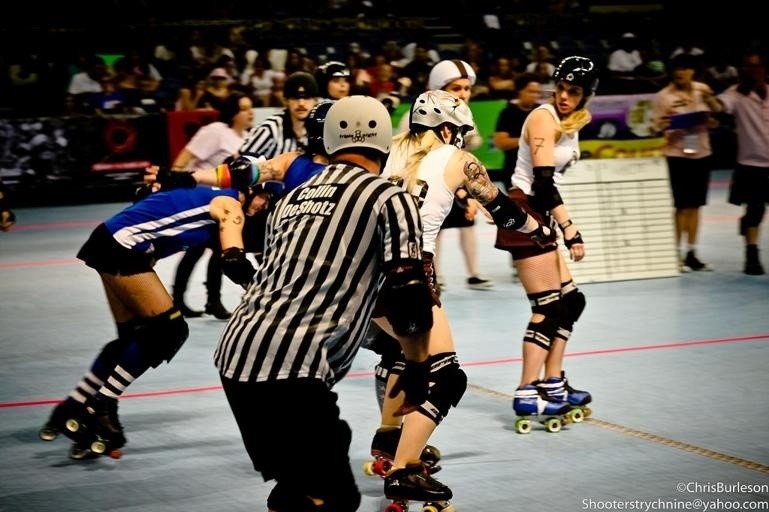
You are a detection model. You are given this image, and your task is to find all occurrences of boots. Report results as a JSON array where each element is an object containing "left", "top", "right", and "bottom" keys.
[
  {"left": 172, "top": 285, "right": 202, "bottom": 317},
  {"left": 202, "top": 283, "right": 232, "bottom": 318}
]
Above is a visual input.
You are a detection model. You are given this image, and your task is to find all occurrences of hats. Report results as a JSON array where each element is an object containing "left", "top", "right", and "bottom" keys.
[{"left": 207, "top": 68, "right": 229, "bottom": 79}]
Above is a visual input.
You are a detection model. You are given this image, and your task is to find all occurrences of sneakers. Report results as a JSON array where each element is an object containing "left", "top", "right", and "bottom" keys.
[
  {"left": 743, "top": 245, "right": 764, "bottom": 275},
  {"left": 681, "top": 250, "right": 714, "bottom": 272},
  {"left": 467, "top": 277, "right": 492, "bottom": 288}
]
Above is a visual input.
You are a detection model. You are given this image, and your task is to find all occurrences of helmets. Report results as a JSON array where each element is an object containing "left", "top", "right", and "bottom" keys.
[
  {"left": 427, "top": 60, "right": 476, "bottom": 89},
  {"left": 305, "top": 101, "right": 332, "bottom": 154},
  {"left": 552, "top": 56, "right": 597, "bottom": 95},
  {"left": 409, "top": 91, "right": 473, "bottom": 129},
  {"left": 230, "top": 154, "right": 285, "bottom": 196},
  {"left": 315, "top": 62, "right": 351, "bottom": 77},
  {"left": 324, "top": 95, "right": 393, "bottom": 155},
  {"left": 282, "top": 72, "right": 320, "bottom": 99}
]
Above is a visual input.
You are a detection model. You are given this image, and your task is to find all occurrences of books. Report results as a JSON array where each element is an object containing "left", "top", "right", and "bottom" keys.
[{"left": 660, "top": 110, "right": 711, "bottom": 130}]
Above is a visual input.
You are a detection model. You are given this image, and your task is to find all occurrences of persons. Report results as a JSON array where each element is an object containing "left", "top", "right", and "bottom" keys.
[
  {"left": 351, "top": 0, "right": 401, "bottom": 97},
  {"left": 398, "top": 54, "right": 482, "bottom": 96},
  {"left": 397, "top": 54, "right": 482, "bottom": 155},
  {"left": 0, "top": 7, "right": 312, "bottom": 118},
  {"left": 39, "top": 180, "right": 291, "bottom": 466},
  {"left": 493, "top": 72, "right": 541, "bottom": 282},
  {"left": 361, "top": 88, "right": 556, "bottom": 511},
  {"left": 168, "top": 89, "right": 255, "bottom": 320},
  {"left": 312, "top": 1, "right": 351, "bottom": 60},
  {"left": 495, "top": 55, "right": 605, "bottom": 435},
  {"left": 648, "top": 47, "right": 720, "bottom": 274},
  {"left": 221, "top": 72, "right": 313, "bottom": 205},
  {"left": 401, "top": 0, "right": 482, "bottom": 55},
  {"left": 482, "top": 1, "right": 736, "bottom": 95},
  {"left": 433, "top": 183, "right": 494, "bottom": 296},
  {"left": 137, "top": 99, "right": 408, "bottom": 430},
  {"left": 213, "top": 91, "right": 434, "bottom": 512},
  {"left": 303, "top": 60, "right": 352, "bottom": 158},
  {"left": 697, "top": 45, "right": 769, "bottom": 275}
]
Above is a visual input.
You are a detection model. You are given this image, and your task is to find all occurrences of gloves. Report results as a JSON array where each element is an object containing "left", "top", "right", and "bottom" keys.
[
  {"left": 557, "top": 223, "right": 586, "bottom": 248},
  {"left": 388, "top": 355, "right": 430, "bottom": 415},
  {"left": 529, "top": 222, "right": 560, "bottom": 248},
  {"left": 143, "top": 164, "right": 196, "bottom": 193}
]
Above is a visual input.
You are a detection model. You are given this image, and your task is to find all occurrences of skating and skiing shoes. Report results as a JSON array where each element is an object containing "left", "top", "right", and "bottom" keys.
[
  {"left": 542, "top": 370, "right": 591, "bottom": 422},
  {"left": 513, "top": 384, "right": 571, "bottom": 434},
  {"left": 66, "top": 397, "right": 126, "bottom": 454},
  {"left": 364, "top": 427, "right": 442, "bottom": 479},
  {"left": 384, "top": 461, "right": 453, "bottom": 512},
  {"left": 39, "top": 399, "right": 98, "bottom": 460}
]
